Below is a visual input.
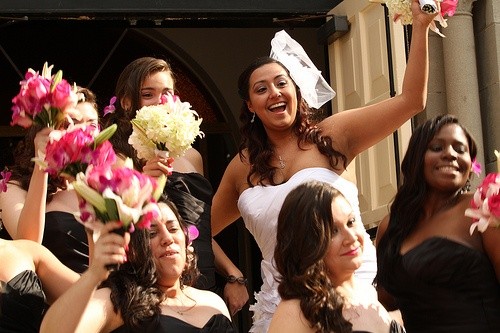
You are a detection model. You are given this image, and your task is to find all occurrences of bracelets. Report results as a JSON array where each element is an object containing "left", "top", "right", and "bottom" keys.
[{"left": 226, "top": 275, "right": 248, "bottom": 285}]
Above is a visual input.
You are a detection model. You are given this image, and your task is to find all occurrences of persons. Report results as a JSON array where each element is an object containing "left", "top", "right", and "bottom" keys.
[
  {"left": 211, "top": 0, "right": 441, "bottom": 332},
  {"left": 374, "top": 113, "right": 500, "bottom": 333},
  {"left": 0, "top": 56, "right": 404, "bottom": 333}
]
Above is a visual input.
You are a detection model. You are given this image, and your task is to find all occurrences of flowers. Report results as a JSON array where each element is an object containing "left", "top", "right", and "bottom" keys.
[
  {"left": 0, "top": 62, "right": 205, "bottom": 271},
  {"left": 386, "top": 0, "right": 458, "bottom": 38},
  {"left": 464, "top": 149, "right": 500, "bottom": 236}
]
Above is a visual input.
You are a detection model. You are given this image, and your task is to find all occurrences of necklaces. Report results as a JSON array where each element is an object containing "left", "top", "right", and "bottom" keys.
[{"left": 275, "top": 140, "right": 293, "bottom": 169}]
